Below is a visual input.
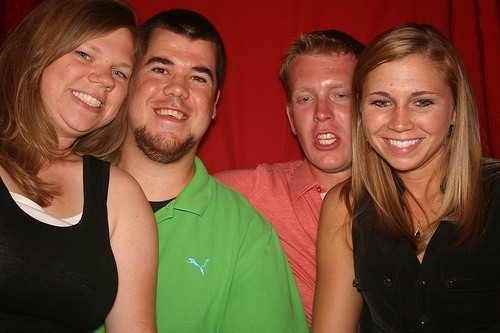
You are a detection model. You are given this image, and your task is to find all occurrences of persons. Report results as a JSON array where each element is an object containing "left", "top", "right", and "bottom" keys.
[
  {"left": 209, "top": 29, "right": 366, "bottom": 333},
  {"left": 0, "top": 0, "right": 159, "bottom": 333},
  {"left": 310, "top": 24, "right": 500, "bottom": 333},
  {"left": 113, "top": 8, "right": 309, "bottom": 333}
]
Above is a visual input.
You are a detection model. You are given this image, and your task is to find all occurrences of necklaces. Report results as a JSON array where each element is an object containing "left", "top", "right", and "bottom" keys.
[{"left": 402, "top": 194, "right": 440, "bottom": 239}]
